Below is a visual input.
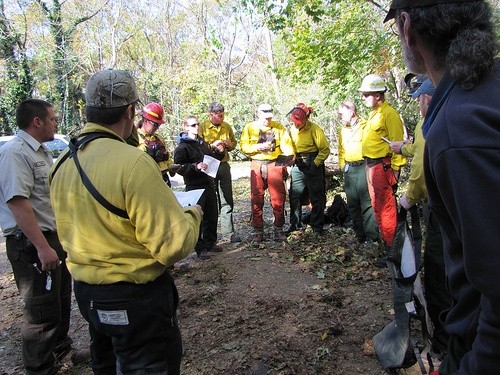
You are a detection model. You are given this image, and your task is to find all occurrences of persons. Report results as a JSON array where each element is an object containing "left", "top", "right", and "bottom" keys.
[
  {"left": 197, "top": 102, "right": 241, "bottom": 242},
  {"left": 0, "top": 100, "right": 93, "bottom": 375},
  {"left": 174, "top": 116, "right": 228, "bottom": 260},
  {"left": 135, "top": 103, "right": 182, "bottom": 188},
  {"left": 384, "top": 1, "right": 499, "bottom": 373},
  {"left": 388, "top": 74, "right": 437, "bottom": 243},
  {"left": 48, "top": 69, "right": 205, "bottom": 375},
  {"left": 286, "top": 102, "right": 331, "bottom": 237},
  {"left": 357, "top": 75, "right": 407, "bottom": 268},
  {"left": 338, "top": 101, "right": 378, "bottom": 251},
  {"left": 240, "top": 103, "right": 288, "bottom": 239}
]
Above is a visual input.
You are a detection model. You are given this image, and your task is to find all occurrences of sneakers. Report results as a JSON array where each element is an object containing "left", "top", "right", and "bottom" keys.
[
  {"left": 174, "top": 262, "right": 189, "bottom": 271},
  {"left": 47, "top": 348, "right": 91, "bottom": 375},
  {"left": 200, "top": 244, "right": 222, "bottom": 260},
  {"left": 223, "top": 227, "right": 282, "bottom": 242},
  {"left": 349, "top": 237, "right": 387, "bottom": 267}
]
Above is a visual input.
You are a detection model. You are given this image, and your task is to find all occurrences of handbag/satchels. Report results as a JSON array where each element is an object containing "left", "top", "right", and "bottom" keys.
[{"left": 369, "top": 204, "right": 422, "bottom": 370}]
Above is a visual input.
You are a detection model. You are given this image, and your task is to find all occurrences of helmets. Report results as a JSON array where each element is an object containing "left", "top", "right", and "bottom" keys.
[
  {"left": 140, "top": 102, "right": 165, "bottom": 124},
  {"left": 356, "top": 75, "right": 386, "bottom": 92}
]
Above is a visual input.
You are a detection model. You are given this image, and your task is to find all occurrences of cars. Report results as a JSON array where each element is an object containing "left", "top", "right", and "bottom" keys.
[{"left": 0, "top": 134, "right": 70, "bottom": 164}]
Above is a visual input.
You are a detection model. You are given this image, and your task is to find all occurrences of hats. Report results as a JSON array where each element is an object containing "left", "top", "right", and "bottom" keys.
[
  {"left": 258, "top": 104, "right": 274, "bottom": 118},
  {"left": 286, "top": 105, "right": 310, "bottom": 124},
  {"left": 407, "top": 75, "right": 435, "bottom": 100},
  {"left": 342, "top": 100, "right": 356, "bottom": 112},
  {"left": 384, "top": 0, "right": 452, "bottom": 22},
  {"left": 209, "top": 102, "right": 224, "bottom": 125},
  {"left": 86, "top": 70, "right": 145, "bottom": 108}
]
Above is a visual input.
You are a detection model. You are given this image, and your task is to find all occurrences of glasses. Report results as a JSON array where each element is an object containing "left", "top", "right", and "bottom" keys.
[
  {"left": 188, "top": 122, "right": 200, "bottom": 127},
  {"left": 147, "top": 119, "right": 161, "bottom": 127},
  {"left": 259, "top": 109, "right": 273, "bottom": 113}
]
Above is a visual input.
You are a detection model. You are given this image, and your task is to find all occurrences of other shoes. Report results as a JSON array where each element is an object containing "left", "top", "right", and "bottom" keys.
[{"left": 284, "top": 225, "right": 326, "bottom": 242}]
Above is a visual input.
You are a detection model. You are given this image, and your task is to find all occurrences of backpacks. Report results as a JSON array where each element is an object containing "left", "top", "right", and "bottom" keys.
[{"left": 325, "top": 194, "right": 351, "bottom": 227}]
